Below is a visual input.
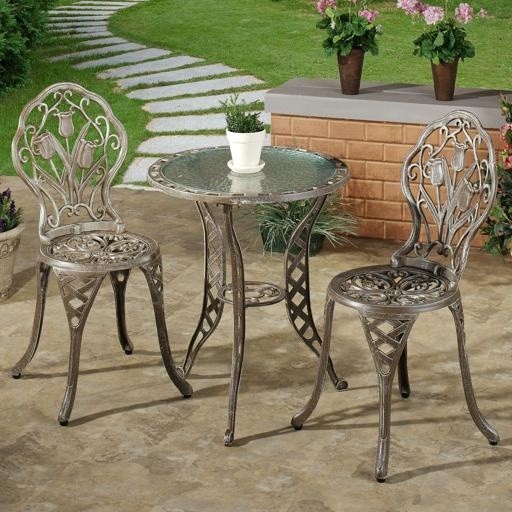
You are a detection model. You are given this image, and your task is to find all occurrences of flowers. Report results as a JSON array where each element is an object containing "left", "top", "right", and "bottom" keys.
[
  {"left": 396, "top": 0, "right": 489, "bottom": 66},
  {"left": 471, "top": 93, "right": 512, "bottom": 266},
  {"left": 316, "top": 0, "right": 385, "bottom": 56},
  {"left": 0, "top": 187, "right": 24, "bottom": 235}
]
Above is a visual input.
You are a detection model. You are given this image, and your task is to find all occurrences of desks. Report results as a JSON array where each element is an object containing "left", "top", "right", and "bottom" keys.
[{"left": 147, "top": 141, "right": 351, "bottom": 446}]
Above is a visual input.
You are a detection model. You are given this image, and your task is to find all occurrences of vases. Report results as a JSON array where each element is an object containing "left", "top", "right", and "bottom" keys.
[
  {"left": 336, "top": 49, "right": 364, "bottom": 96},
  {"left": 430, "top": 57, "right": 459, "bottom": 101},
  {"left": 1, "top": 223, "right": 25, "bottom": 302}
]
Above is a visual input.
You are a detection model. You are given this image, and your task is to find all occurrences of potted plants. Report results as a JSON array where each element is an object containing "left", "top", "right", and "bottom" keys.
[
  {"left": 257, "top": 190, "right": 359, "bottom": 257},
  {"left": 218, "top": 93, "right": 267, "bottom": 174}
]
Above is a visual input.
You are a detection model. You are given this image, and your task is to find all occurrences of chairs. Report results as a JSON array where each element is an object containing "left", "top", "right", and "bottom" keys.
[
  {"left": 11, "top": 81, "right": 194, "bottom": 426},
  {"left": 291, "top": 108, "right": 500, "bottom": 483}
]
